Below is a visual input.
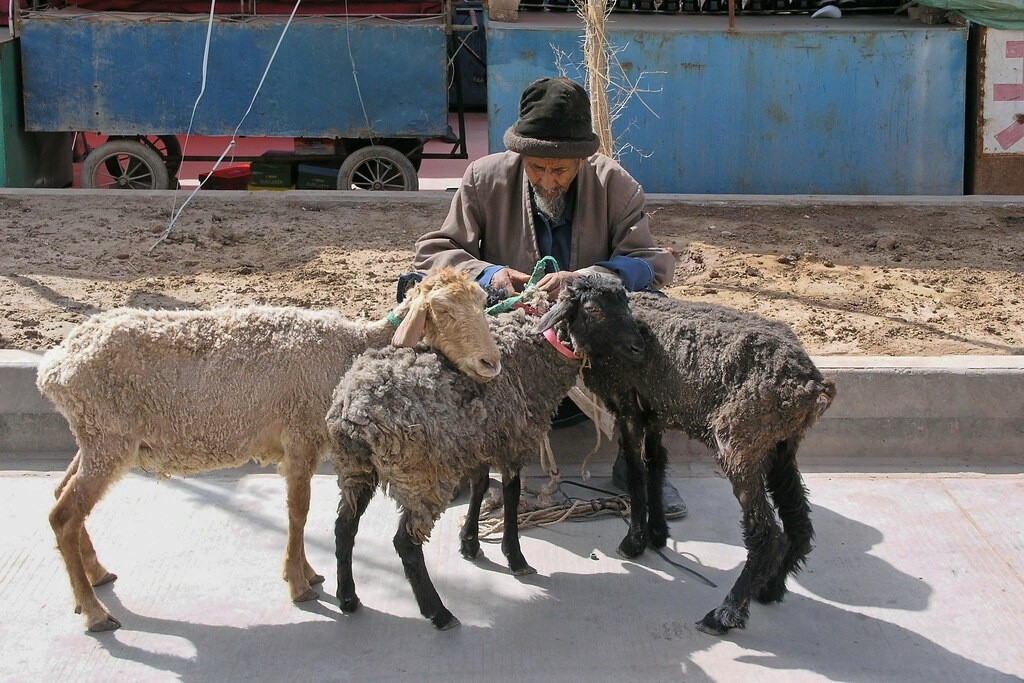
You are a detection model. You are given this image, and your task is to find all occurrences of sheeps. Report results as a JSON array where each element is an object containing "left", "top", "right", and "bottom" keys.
[{"left": 28, "top": 261, "right": 840, "bottom": 637}]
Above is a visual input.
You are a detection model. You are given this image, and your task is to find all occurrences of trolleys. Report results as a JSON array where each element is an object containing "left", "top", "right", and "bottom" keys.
[{"left": 17, "top": 5, "right": 473, "bottom": 192}]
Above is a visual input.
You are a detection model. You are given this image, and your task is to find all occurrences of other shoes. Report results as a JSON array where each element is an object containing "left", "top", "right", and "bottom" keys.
[{"left": 612, "top": 446, "right": 688, "bottom": 521}]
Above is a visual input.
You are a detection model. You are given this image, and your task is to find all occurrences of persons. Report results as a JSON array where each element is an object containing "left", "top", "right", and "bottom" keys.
[{"left": 414, "top": 76, "right": 687, "bottom": 518}]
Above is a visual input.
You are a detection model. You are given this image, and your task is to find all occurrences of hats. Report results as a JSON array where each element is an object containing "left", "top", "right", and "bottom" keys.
[{"left": 502, "top": 79, "right": 601, "bottom": 158}]
